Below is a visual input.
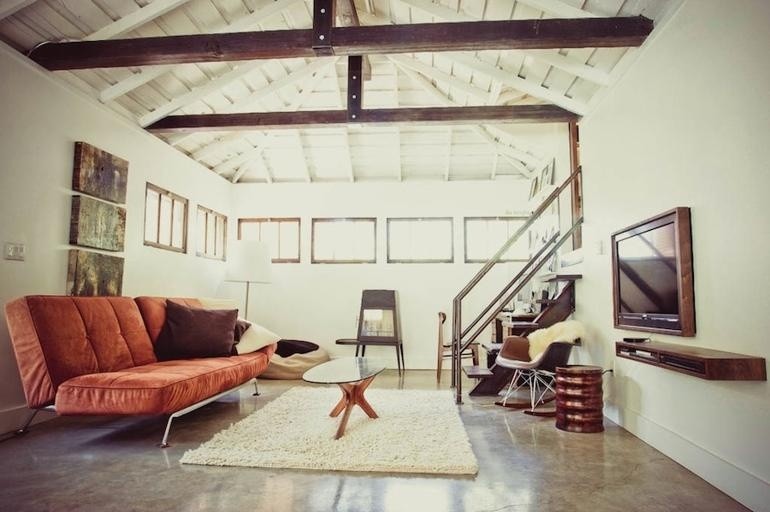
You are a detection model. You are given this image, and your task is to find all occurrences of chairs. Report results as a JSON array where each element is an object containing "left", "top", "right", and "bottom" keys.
[
  {"left": 494, "top": 320, "right": 585, "bottom": 418},
  {"left": 437, "top": 311, "right": 480, "bottom": 383}
]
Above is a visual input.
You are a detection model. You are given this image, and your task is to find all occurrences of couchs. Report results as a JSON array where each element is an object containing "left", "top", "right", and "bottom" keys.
[{"left": 1, "top": 294, "right": 278, "bottom": 447}]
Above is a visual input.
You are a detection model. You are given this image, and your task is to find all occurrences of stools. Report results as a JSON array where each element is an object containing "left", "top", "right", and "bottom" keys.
[{"left": 254, "top": 338, "right": 332, "bottom": 382}]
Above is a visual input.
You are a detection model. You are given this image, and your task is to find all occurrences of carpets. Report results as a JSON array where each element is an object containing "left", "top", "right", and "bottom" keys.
[{"left": 180, "top": 387, "right": 478, "bottom": 476}]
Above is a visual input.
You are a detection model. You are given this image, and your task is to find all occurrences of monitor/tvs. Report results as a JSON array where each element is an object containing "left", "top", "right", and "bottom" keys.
[{"left": 615, "top": 214, "right": 680, "bottom": 331}]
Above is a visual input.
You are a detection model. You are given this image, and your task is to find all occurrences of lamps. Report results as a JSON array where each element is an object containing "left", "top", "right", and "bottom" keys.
[{"left": 224, "top": 238, "right": 278, "bottom": 320}]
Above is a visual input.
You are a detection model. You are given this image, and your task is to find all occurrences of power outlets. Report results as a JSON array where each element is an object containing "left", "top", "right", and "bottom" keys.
[{"left": 610, "top": 360, "right": 615, "bottom": 375}]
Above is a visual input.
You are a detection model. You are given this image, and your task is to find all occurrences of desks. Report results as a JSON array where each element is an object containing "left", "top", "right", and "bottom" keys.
[
  {"left": 336, "top": 337, "right": 406, "bottom": 376},
  {"left": 556, "top": 364, "right": 603, "bottom": 433},
  {"left": 490, "top": 310, "right": 539, "bottom": 344}
]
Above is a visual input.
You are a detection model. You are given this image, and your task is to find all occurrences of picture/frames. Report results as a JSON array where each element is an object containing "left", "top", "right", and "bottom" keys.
[
  {"left": 528, "top": 177, "right": 538, "bottom": 197},
  {"left": 541, "top": 159, "right": 555, "bottom": 192}
]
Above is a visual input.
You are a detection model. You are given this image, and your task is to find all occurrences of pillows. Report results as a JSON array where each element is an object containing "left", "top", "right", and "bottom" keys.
[
  {"left": 232, "top": 320, "right": 251, "bottom": 355},
  {"left": 235, "top": 320, "right": 282, "bottom": 354},
  {"left": 156, "top": 299, "right": 239, "bottom": 359}
]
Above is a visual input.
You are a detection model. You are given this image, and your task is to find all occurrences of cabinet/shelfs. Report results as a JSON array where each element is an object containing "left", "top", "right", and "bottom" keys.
[{"left": 614, "top": 340, "right": 768, "bottom": 384}]
